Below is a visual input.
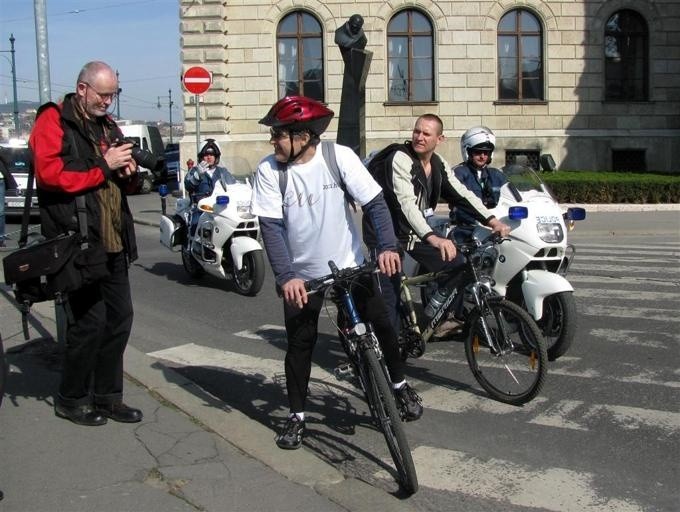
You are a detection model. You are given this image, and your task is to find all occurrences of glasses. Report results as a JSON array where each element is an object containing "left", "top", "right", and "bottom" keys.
[
  {"left": 470, "top": 147, "right": 492, "bottom": 155},
  {"left": 84, "top": 82, "right": 121, "bottom": 103},
  {"left": 269, "top": 128, "right": 288, "bottom": 138}
]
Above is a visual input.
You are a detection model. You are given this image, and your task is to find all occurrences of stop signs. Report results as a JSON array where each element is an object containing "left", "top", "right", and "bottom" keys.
[{"left": 182, "top": 66, "right": 211, "bottom": 96}]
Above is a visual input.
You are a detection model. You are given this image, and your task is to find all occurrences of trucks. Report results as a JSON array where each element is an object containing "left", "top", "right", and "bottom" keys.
[
  {"left": 0, "top": 134, "right": 40, "bottom": 217},
  {"left": 118, "top": 125, "right": 168, "bottom": 194}
]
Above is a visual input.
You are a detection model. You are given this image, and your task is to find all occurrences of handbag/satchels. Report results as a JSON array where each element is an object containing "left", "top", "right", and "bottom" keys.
[{"left": 2, "top": 232, "right": 85, "bottom": 306}]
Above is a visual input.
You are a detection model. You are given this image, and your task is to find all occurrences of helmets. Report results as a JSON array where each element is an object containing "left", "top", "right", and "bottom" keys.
[
  {"left": 257, "top": 96, "right": 334, "bottom": 136},
  {"left": 196, "top": 139, "right": 222, "bottom": 164},
  {"left": 461, "top": 125, "right": 496, "bottom": 165}
]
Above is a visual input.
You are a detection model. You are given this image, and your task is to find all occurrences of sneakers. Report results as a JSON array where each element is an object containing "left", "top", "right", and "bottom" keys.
[
  {"left": 394, "top": 383, "right": 423, "bottom": 420},
  {"left": 435, "top": 315, "right": 466, "bottom": 338},
  {"left": 278, "top": 412, "right": 305, "bottom": 450}
]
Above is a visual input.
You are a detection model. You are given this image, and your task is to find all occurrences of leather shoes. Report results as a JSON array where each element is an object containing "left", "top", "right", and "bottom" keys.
[
  {"left": 55, "top": 399, "right": 106, "bottom": 425},
  {"left": 93, "top": 399, "right": 144, "bottom": 422}
]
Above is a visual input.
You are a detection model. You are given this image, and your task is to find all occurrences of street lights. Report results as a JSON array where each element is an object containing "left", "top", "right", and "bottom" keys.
[{"left": 156, "top": 89, "right": 173, "bottom": 143}]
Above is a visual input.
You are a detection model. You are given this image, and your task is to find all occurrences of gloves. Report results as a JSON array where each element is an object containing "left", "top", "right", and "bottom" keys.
[{"left": 190, "top": 160, "right": 210, "bottom": 182}]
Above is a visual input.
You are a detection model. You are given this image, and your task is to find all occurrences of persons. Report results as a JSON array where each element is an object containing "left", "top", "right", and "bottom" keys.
[
  {"left": 27, "top": 62, "right": 142, "bottom": 426},
  {"left": 248, "top": 96, "right": 402, "bottom": 450},
  {"left": 362, "top": 113, "right": 511, "bottom": 319},
  {"left": 184, "top": 142, "right": 239, "bottom": 237},
  {"left": 446, "top": 126, "right": 510, "bottom": 230},
  {"left": 0, "top": 158, "right": 18, "bottom": 248}
]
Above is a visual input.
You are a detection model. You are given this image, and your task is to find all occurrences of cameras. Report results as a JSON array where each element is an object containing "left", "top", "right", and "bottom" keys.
[{"left": 115, "top": 134, "right": 168, "bottom": 172}]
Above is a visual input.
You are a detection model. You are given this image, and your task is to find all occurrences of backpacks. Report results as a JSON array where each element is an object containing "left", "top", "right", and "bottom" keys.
[{"left": 367, "top": 140, "right": 421, "bottom": 209}]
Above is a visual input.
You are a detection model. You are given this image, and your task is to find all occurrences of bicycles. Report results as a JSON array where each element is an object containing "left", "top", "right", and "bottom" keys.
[
  {"left": 284, "top": 247, "right": 418, "bottom": 495},
  {"left": 337, "top": 228, "right": 550, "bottom": 405}
]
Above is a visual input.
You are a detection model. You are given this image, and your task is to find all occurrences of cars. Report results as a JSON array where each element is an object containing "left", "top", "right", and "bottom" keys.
[
  {"left": 166, "top": 143, "right": 178, "bottom": 152},
  {"left": 165, "top": 150, "right": 179, "bottom": 176}
]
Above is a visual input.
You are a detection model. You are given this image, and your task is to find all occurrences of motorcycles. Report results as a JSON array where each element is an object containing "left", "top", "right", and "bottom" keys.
[
  {"left": 158, "top": 158, "right": 265, "bottom": 297},
  {"left": 375, "top": 164, "right": 586, "bottom": 361}
]
